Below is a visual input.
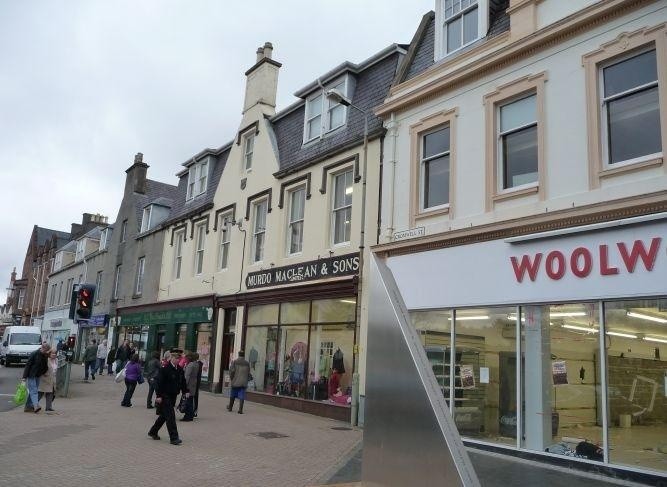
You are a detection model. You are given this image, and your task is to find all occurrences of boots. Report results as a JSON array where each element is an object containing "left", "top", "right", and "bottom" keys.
[
  {"left": 227, "top": 397, "right": 235, "bottom": 411},
  {"left": 238, "top": 399, "right": 243, "bottom": 413}
]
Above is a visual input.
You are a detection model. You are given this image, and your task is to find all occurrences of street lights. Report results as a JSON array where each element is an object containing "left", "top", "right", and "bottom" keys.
[{"left": 325, "top": 88, "right": 370, "bottom": 432}]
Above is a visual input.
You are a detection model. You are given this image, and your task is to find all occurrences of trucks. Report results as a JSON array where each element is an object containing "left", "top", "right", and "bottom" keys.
[{"left": 0, "top": 326, "right": 42, "bottom": 367}]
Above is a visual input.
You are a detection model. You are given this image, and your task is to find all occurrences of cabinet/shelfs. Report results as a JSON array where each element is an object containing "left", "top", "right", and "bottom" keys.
[
  {"left": 420, "top": 329, "right": 486, "bottom": 437},
  {"left": 594, "top": 355, "right": 666, "bottom": 428}
]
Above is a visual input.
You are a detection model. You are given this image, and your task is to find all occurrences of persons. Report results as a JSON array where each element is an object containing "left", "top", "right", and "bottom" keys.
[
  {"left": 145, "top": 346, "right": 183, "bottom": 445},
  {"left": 21, "top": 335, "right": 203, "bottom": 421},
  {"left": 225, "top": 350, "right": 250, "bottom": 413},
  {"left": 333, "top": 347, "right": 344, "bottom": 384}
]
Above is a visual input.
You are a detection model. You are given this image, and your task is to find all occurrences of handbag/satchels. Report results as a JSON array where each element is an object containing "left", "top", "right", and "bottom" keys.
[
  {"left": 138, "top": 375, "right": 144, "bottom": 384},
  {"left": 115, "top": 368, "right": 126, "bottom": 383}
]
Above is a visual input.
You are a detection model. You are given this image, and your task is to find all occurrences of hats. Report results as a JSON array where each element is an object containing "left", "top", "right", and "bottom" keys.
[{"left": 170, "top": 349, "right": 183, "bottom": 356}]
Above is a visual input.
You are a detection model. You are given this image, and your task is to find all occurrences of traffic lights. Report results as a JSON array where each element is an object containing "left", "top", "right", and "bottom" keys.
[{"left": 76, "top": 283, "right": 97, "bottom": 320}]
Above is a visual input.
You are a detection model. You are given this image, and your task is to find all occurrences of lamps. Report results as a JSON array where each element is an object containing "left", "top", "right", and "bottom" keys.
[{"left": 447, "top": 307, "right": 667, "bottom": 347}]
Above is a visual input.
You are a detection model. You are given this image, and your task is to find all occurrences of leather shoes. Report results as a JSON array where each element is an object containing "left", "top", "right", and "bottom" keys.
[{"left": 24, "top": 406, "right": 54, "bottom": 413}]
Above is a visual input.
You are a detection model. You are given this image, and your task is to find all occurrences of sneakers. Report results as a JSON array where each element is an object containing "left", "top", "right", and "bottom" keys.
[
  {"left": 180, "top": 417, "right": 193, "bottom": 421},
  {"left": 170, "top": 439, "right": 182, "bottom": 445},
  {"left": 148, "top": 431, "right": 160, "bottom": 440}
]
